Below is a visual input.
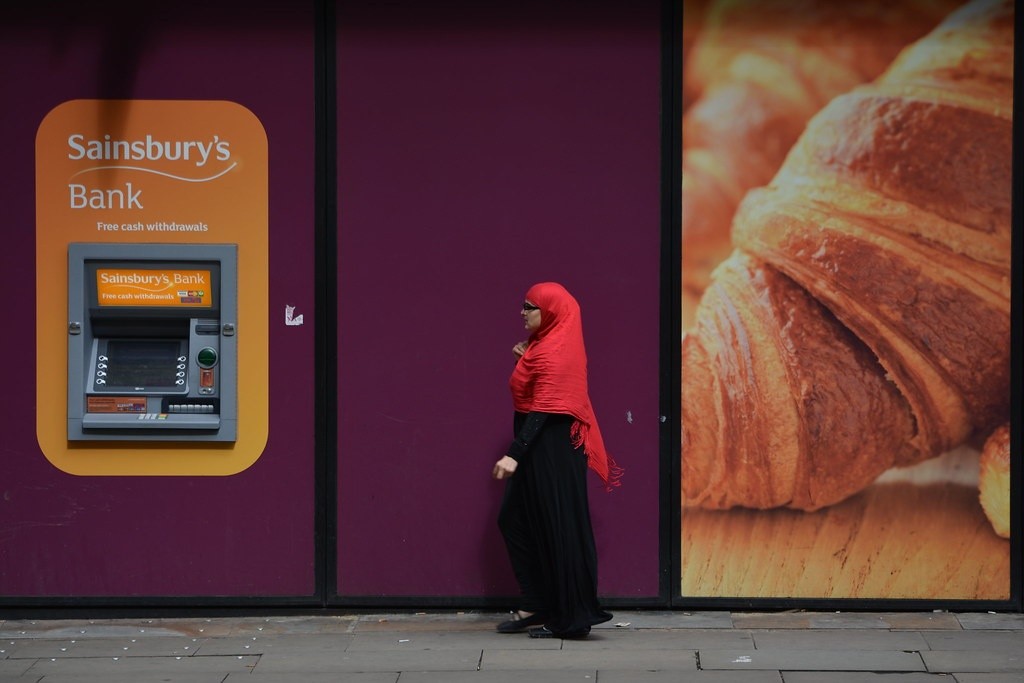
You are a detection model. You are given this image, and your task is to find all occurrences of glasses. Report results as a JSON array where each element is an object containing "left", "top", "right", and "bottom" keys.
[{"left": 523, "top": 303, "right": 541, "bottom": 311}]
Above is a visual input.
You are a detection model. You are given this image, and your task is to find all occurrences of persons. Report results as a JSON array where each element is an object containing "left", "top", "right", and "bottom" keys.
[{"left": 494, "top": 282, "right": 624, "bottom": 639}]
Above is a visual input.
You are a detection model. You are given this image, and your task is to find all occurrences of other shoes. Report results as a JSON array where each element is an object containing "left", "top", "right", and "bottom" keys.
[
  {"left": 497, "top": 610, "right": 545, "bottom": 633},
  {"left": 527, "top": 621, "right": 591, "bottom": 638}
]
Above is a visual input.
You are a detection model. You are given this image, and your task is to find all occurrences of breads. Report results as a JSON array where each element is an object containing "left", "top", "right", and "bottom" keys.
[{"left": 681, "top": 0, "right": 1015, "bottom": 542}]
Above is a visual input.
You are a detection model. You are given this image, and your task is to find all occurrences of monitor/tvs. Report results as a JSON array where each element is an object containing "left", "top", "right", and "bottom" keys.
[{"left": 105, "top": 341, "right": 180, "bottom": 386}]
